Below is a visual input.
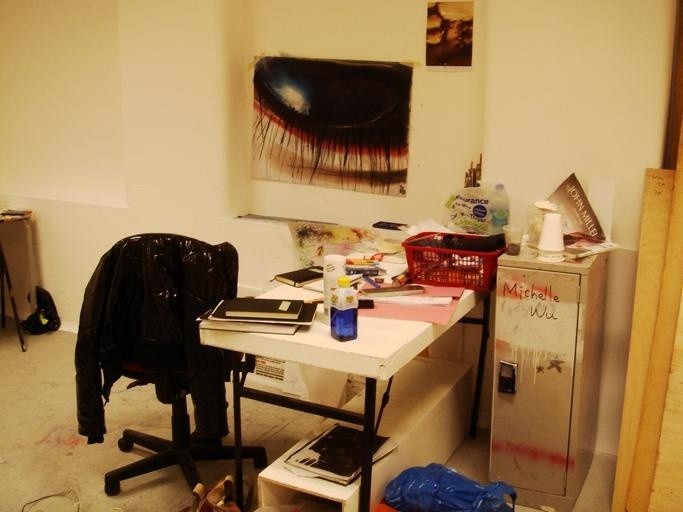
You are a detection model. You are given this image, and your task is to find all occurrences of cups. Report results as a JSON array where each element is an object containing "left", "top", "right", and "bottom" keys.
[
  {"left": 537, "top": 212, "right": 566, "bottom": 264},
  {"left": 503, "top": 225, "right": 523, "bottom": 255}
]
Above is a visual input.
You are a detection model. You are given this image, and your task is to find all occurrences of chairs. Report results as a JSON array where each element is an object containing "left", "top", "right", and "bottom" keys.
[{"left": 102, "top": 235, "right": 269, "bottom": 505}]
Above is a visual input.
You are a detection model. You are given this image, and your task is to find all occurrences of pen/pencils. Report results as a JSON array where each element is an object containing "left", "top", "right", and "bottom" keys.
[
  {"left": 465, "top": 152, "right": 482, "bottom": 187},
  {"left": 363, "top": 274, "right": 380, "bottom": 289}
]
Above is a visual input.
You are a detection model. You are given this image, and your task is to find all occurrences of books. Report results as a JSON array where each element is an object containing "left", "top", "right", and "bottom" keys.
[
  {"left": 208, "top": 300, "right": 318, "bottom": 325},
  {"left": 563, "top": 232, "right": 619, "bottom": 259},
  {"left": 284, "top": 422, "right": 399, "bottom": 486},
  {"left": 303, "top": 274, "right": 363, "bottom": 293},
  {"left": 275, "top": 265, "right": 323, "bottom": 288},
  {"left": 195, "top": 309, "right": 301, "bottom": 335},
  {"left": 225, "top": 298, "right": 304, "bottom": 319}
]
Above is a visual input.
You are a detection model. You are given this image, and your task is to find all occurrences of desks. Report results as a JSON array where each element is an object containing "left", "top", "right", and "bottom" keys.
[
  {"left": 199, "top": 248, "right": 495, "bottom": 512},
  {"left": 0, "top": 210, "right": 34, "bottom": 353}
]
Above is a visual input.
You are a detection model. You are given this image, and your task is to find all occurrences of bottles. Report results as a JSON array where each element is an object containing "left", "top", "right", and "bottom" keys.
[
  {"left": 329, "top": 276, "right": 358, "bottom": 342},
  {"left": 323, "top": 254, "right": 347, "bottom": 315},
  {"left": 491, "top": 183, "right": 510, "bottom": 230}
]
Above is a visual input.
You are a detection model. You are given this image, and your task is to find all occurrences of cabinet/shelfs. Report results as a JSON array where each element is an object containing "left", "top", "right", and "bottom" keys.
[{"left": 489, "top": 242, "right": 610, "bottom": 512}]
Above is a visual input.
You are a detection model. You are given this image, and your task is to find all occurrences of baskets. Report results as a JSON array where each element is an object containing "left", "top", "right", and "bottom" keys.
[{"left": 403, "top": 227, "right": 506, "bottom": 291}]
